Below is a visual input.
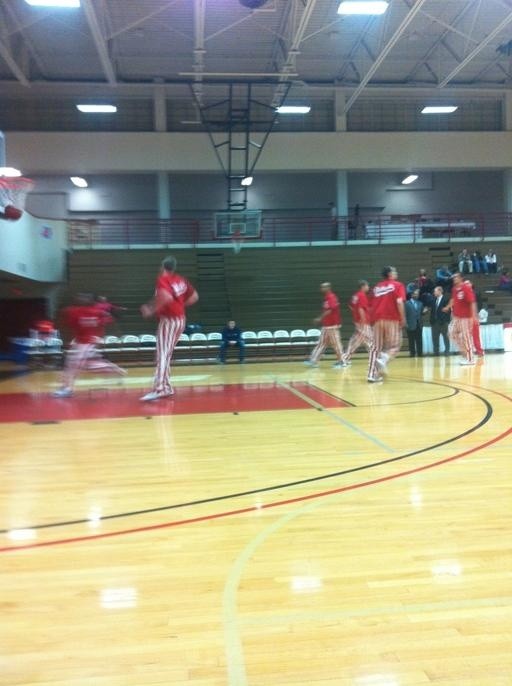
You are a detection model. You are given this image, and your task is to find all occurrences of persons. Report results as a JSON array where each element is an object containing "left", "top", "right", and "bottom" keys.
[
  {"left": 366, "top": 265, "right": 408, "bottom": 383},
  {"left": 138, "top": 255, "right": 200, "bottom": 401},
  {"left": 302, "top": 281, "right": 347, "bottom": 369},
  {"left": 91, "top": 295, "right": 128, "bottom": 314},
  {"left": 339, "top": 279, "right": 374, "bottom": 366},
  {"left": 52, "top": 291, "right": 130, "bottom": 399},
  {"left": 215, "top": 319, "right": 247, "bottom": 366},
  {"left": 35, "top": 320, "right": 56, "bottom": 339},
  {"left": 403, "top": 247, "right": 512, "bottom": 366},
  {"left": 327, "top": 201, "right": 338, "bottom": 240}
]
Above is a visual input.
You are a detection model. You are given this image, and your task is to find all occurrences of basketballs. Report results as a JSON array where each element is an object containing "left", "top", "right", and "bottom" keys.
[{"left": 6, "top": 205, "right": 22, "bottom": 219}]
